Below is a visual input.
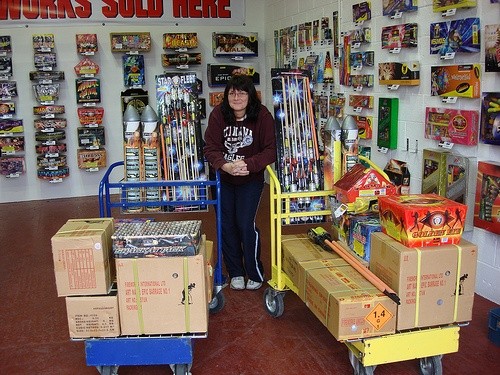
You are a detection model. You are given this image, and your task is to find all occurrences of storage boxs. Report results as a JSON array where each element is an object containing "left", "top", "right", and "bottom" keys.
[
  {"left": 51, "top": 217, "right": 114, "bottom": 298},
  {"left": 1, "top": 32, "right": 258, "bottom": 260},
  {"left": 270, "top": 66, "right": 326, "bottom": 228},
  {"left": 65, "top": 297, "right": 120, "bottom": 338},
  {"left": 115, "top": 234, "right": 208, "bottom": 335},
  {"left": 276, "top": 234, "right": 369, "bottom": 303},
  {"left": 305, "top": 265, "right": 397, "bottom": 341},
  {"left": 328, "top": 1, "right": 499, "bottom": 264},
  {"left": 371, "top": 232, "right": 478, "bottom": 331},
  {"left": 206, "top": 241, "right": 215, "bottom": 303}
]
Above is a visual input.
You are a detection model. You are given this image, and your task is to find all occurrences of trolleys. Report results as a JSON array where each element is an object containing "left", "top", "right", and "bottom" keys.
[
  {"left": 70, "top": 161, "right": 229, "bottom": 375},
  {"left": 266, "top": 155, "right": 470, "bottom": 375}
]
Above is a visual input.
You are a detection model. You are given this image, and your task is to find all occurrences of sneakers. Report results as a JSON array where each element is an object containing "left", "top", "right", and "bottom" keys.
[
  {"left": 246, "top": 278, "right": 262, "bottom": 289},
  {"left": 230, "top": 276, "right": 245, "bottom": 289}
]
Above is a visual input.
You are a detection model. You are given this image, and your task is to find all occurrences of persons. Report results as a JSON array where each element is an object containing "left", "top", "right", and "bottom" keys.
[{"left": 204, "top": 74, "right": 278, "bottom": 289}]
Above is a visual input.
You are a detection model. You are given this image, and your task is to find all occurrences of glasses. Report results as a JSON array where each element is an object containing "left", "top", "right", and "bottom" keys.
[{"left": 227, "top": 92, "right": 248, "bottom": 97}]
